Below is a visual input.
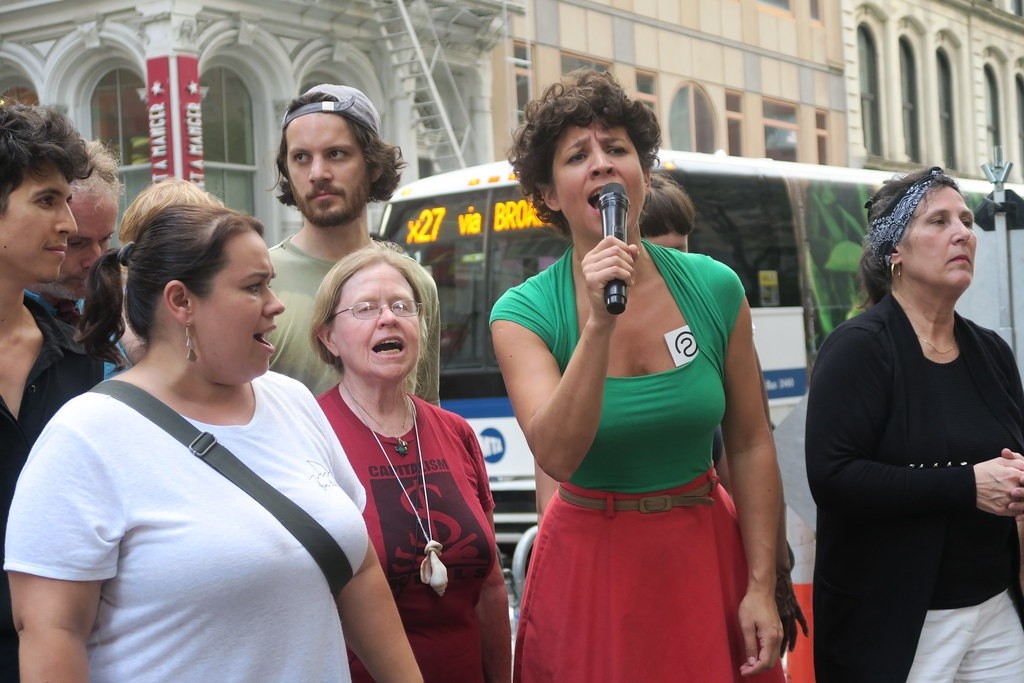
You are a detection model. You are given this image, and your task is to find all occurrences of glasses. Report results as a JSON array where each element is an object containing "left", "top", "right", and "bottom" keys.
[{"left": 330, "top": 299, "right": 422, "bottom": 320}]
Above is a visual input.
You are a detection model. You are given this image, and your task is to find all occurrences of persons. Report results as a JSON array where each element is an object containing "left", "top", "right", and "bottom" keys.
[
  {"left": 259, "top": 82, "right": 445, "bottom": 406},
  {"left": 3, "top": 175, "right": 427, "bottom": 683},
  {"left": 22, "top": 133, "right": 146, "bottom": 383},
  {"left": 0, "top": 95, "right": 104, "bottom": 683},
  {"left": 309, "top": 238, "right": 514, "bottom": 683},
  {"left": 802, "top": 165, "right": 1024, "bottom": 683},
  {"left": 533, "top": 169, "right": 810, "bottom": 658},
  {"left": 489, "top": 64, "right": 792, "bottom": 683}
]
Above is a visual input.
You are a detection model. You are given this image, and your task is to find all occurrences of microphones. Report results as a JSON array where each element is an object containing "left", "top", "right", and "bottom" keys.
[{"left": 597, "top": 182, "right": 631, "bottom": 315}]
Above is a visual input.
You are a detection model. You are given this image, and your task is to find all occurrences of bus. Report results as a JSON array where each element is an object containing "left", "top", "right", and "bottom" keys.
[{"left": 377, "top": 148, "right": 1024, "bottom": 683}]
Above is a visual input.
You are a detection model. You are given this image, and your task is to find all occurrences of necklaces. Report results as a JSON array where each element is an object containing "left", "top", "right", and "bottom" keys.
[
  {"left": 916, "top": 334, "right": 957, "bottom": 355},
  {"left": 341, "top": 377, "right": 412, "bottom": 456},
  {"left": 347, "top": 388, "right": 449, "bottom": 596}
]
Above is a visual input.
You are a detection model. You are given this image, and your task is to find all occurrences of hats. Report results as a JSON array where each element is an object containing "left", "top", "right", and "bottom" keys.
[{"left": 282, "top": 83, "right": 381, "bottom": 141}]
